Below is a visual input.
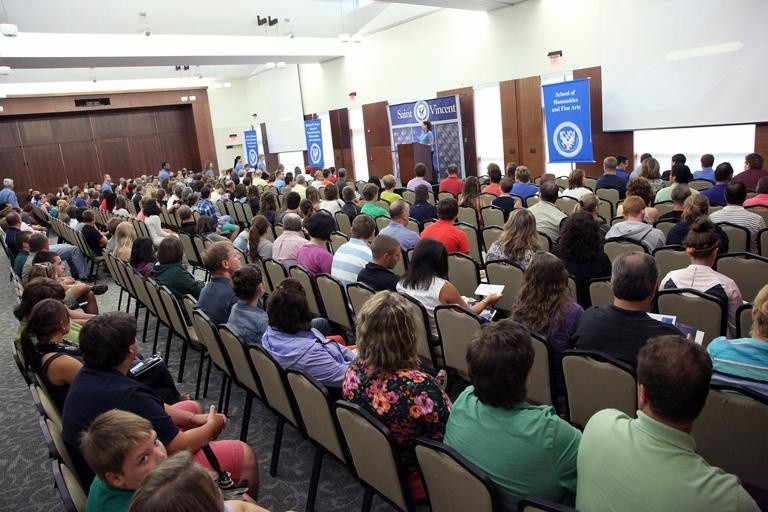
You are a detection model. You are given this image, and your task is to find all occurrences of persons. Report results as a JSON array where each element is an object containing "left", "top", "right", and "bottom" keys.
[
  {"left": 223, "top": 180, "right": 236, "bottom": 203},
  {"left": 198, "top": 240, "right": 266, "bottom": 327},
  {"left": 595, "top": 157, "right": 626, "bottom": 200},
  {"left": 562, "top": 169, "right": 593, "bottom": 200},
  {"left": 0, "top": 208, "right": 46, "bottom": 240},
  {"left": 0, "top": 202, "right": 14, "bottom": 213},
  {"left": 21, "top": 205, "right": 48, "bottom": 238},
  {"left": 21, "top": 234, "right": 109, "bottom": 315},
  {"left": 272, "top": 213, "right": 309, "bottom": 277},
  {"left": 107, "top": 217, "right": 123, "bottom": 253},
  {"left": 485, "top": 210, "right": 540, "bottom": 273},
  {"left": 159, "top": 162, "right": 171, "bottom": 188},
  {"left": 707, "top": 283, "right": 768, "bottom": 404},
  {"left": 33, "top": 250, "right": 103, "bottom": 325},
  {"left": 574, "top": 251, "right": 687, "bottom": 372},
  {"left": 380, "top": 174, "right": 404, "bottom": 203},
  {"left": 638, "top": 157, "right": 665, "bottom": 197},
  {"left": 419, "top": 199, "right": 470, "bottom": 257},
  {"left": 233, "top": 226, "right": 251, "bottom": 265},
  {"left": 489, "top": 178, "right": 523, "bottom": 225},
  {"left": 31, "top": 191, "right": 40, "bottom": 205},
  {"left": 13, "top": 231, "right": 31, "bottom": 282},
  {"left": 342, "top": 290, "right": 455, "bottom": 507},
  {"left": 442, "top": 319, "right": 584, "bottom": 512},
  {"left": 297, "top": 213, "right": 336, "bottom": 314},
  {"left": 409, "top": 185, "right": 438, "bottom": 229},
  {"left": 14, "top": 277, "right": 84, "bottom": 348},
  {"left": 654, "top": 164, "right": 700, "bottom": 205},
  {"left": 659, "top": 184, "right": 692, "bottom": 219},
  {"left": 666, "top": 194, "right": 729, "bottom": 270},
  {"left": 234, "top": 155, "right": 243, "bottom": 172},
  {"left": 57, "top": 200, "right": 69, "bottom": 222},
  {"left": 179, "top": 205, "right": 201, "bottom": 238},
  {"left": 336, "top": 168, "right": 349, "bottom": 202},
  {"left": 275, "top": 164, "right": 284, "bottom": 179},
  {"left": 457, "top": 177, "right": 487, "bottom": 231},
  {"left": 731, "top": 154, "right": 768, "bottom": 192},
  {"left": 310, "top": 170, "right": 324, "bottom": 192},
  {"left": 81, "top": 210, "right": 111, "bottom": 274},
  {"left": 195, "top": 216, "right": 229, "bottom": 242},
  {"left": 205, "top": 161, "right": 215, "bottom": 176},
  {"left": 510, "top": 251, "right": 585, "bottom": 405},
  {"left": 136, "top": 197, "right": 150, "bottom": 221},
  {"left": 0, "top": 178, "right": 19, "bottom": 209},
  {"left": 26, "top": 263, "right": 58, "bottom": 282},
  {"left": 273, "top": 170, "right": 286, "bottom": 194},
  {"left": 113, "top": 197, "right": 134, "bottom": 217},
  {"left": 744, "top": 176, "right": 768, "bottom": 210},
  {"left": 361, "top": 183, "right": 391, "bottom": 222},
  {"left": 228, "top": 264, "right": 328, "bottom": 343},
  {"left": 252, "top": 173, "right": 268, "bottom": 190},
  {"left": 259, "top": 190, "right": 282, "bottom": 228},
  {"left": 701, "top": 162, "right": 734, "bottom": 208},
  {"left": 129, "top": 450, "right": 270, "bottom": 512},
  {"left": 482, "top": 170, "right": 502, "bottom": 198},
  {"left": 330, "top": 215, "right": 376, "bottom": 318},
  {"left": 279, "top": 172, "right": 293, "bottom": 212},
  {"left": 143, "top": 199, "right": 181, "bottom": 246},
  {"left": 439, "top": 164, "right": 464, "bottom": 223},
  {"left": 301, "top": 165, "right": 314, "bottom": 185},
  {"left": 66, "top": 205, "right": 80, "bottom": 229},
  {"left": 569, "top": 193, "right": 611, "bottom": 251},
  {"left": 396, "top": 238, "right": 503, "bottom": 357},
  {"left": 55, "top": 180, "right": 117, "bottom": 210},
  {"left": 35, "top": 194, "right": 43, "bottom": 208},
  {"left": 662, "top": 154, "right": 687, "bottom": 181},
  {"left": 50, "top": 196, "right": 60, "bottom": 218},
  {"left": 320, "top": 169, "right": 333, "bottom": 187},
  {"left": 535, "top": 173, "right": 563, "bottom": 197},
  {"left": 177, "top": 186, "right": 192, "bottom": 206},
  {"left": 113, "top": 222, "right": 137, "bottom": 263},
  {"left": 150, "top": 235, "right": 206, "bottom": 327},
  {"left": 629, "top": 153, "right": 652, "bottom": 179},
  {"left": 129, "top": 237, "right": 156, "bottom": 280},
  {"left": 208, "top": 183, "right": 225, "bottom": 214},
  {"left": 189, "top": 192, "right": 232, "bottom": 243},
  {"left": 102, "top": 174, "right": 113, "bottom": 193},
  {"left": 406, "top": 164, "right": 433, "bottom": 193},
  {"left": 421, "top": 121, "right": 438, "bottom": 184},
  {"left": 171, "top": 168, "right": 258, "bottom": 191},
  {"left": 616, "top": 177, "right": 661, "bottom": 229},
  {"left": 74, "top": 207, "right": 112, "bottom": 240},
  {"left": 341, "top": 186, "right": 359, "bottom": 226},
  {"left": 320, "top": 184, "right": 346, "bottom": 231},
  {"left": 111, "top": 175, "right": 176, "bottom": 215},
  {"left": 693, "top": 154, "right": 715, "bottom": 182},
  {"left": 41, "top": 193, "right": 55, "bottom": 221},
  {"left": 483, "top": 164, "right": 500, "bottom": 184},
  {"left": 577, "top": 335, "right": 763, "bottom": 511},
  {"left": 250, "top": 215, "right": 272, "bottom": 261},
  {"left": 167, "top": 182, "right": 186, "bottom": 211},
  {"left": 378, "top": 200, "right": 421, "bottom": 252},
  {"left": 258, "top": 154, "right": 270, "bottom": 180},
  {"left": 708, "top": 182, "right": 767, "bottom": 256},
  {"left": 357, "top": 235, "right": 402, "bottom": 295},
  {"left": 261, "top": 277, "right": 361, "bottom": 401},
  {"left": 79, "top": 411, "right": 259, "bottom": 512},
  {"left": 504, "top": 162, "right": 517, "bottom": 184},
  {"left": 246, "top": 185, "right": 260, "bottom": 218},
  {"left": 199, "top": 188, "right": 240, "bottom": 242},
  {"left": 604, "top": 196, "right": 667, "bottom": 256},
  {"left": 19, "top": 299, "right": 193, "bottom": 415},
  {"left": 294, "top": 167, "right": 302, "bottom": 180},
  {"left": 278, "top": 192, "right": 302, "bottom": 225},
  {"left": 510, "top": 166, "right": 539, "bottom": 203},
  {"left": 28, "top": 188, "right": 33, "bottom": 201},
  {"left": 660, "top": 216, "right": 743, "bottom": 340},
  {"left": 291, "top": 175, "right": 307, "bottom": 212},
  {"left": 328, "top": 166, "right": 337, "bottom": 183},
  {"left": 299, "top": 200, "right": 318, "bottom": 235},
  {"left": 61, "top": 312, "right": 258, "bottom": 502},
  {"left": 360, "top": 176, "right": 382, "bottom": 201},
  {"left": 615, "top": 155, "right": 630, "bottom": 184},
  {"left": 550, "top": 211, "right": 612, "bottom": 310},
  {"left": 305, "top": 186, "right": 320, "bottom": 212},
  {"left": 525, "top": 181, "right": 568, "bottom": 247},
  {"left": 4, "top": 212, "right": 101, "bottom": 282},
  {"left": 231, "top": 184, "right": 246, "bottom": 204}
]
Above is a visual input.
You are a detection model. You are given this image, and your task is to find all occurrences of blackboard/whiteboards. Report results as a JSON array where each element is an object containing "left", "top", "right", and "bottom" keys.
[{"left": 633, "top": 122, "right": 757, "bottom": 178}]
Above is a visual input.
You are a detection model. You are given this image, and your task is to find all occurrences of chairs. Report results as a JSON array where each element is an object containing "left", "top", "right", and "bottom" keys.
[
  {"left": 716, "top": 221, "right": 750, "bottom": 258},
  {"left": 516, "top": 491, "right": 587, "bottom": 512},
  {"left": 131, "top": 270, "right": 160, "bottom": 343},
  {"left": 403, "top": 191, "right": 418, "bottom": 204},
  {"left": 123, "top": 216, "right": 151, "bottom": 244},
  {"left": 716, "top": 253, "right": 768, "bottom": 303},
  {"left": 479, "top": 194, "right": 500, "bottom": 208},
  {"left": 11, "top": 340, "right": 52, "bottom": 393},
  {"left": 375, "top": 213, "right": 390, "bottom": 233},
  {"left": 81, "top": 206, "right": 125, "bottom": 235},
  {"left": 168, "top": 209, "right": 178, "bottom": 227},
  {"left": 0, "top": 232, "right": 16, "bottom": 281},
  {"left": 745, "top": 201, "right": 768, "bottom": 225},
  {"left": 192, "top": 232, "right": 211, "bottom": 279},
  {"left": 271, "top": 223, "right": 283, "bottom": 237},
  {"left": 264, "top": 258, "right": 287, "bottom": 290},
  {"left": 233, "top": 200, "right": 247, "bottom": 226},
  {"left": 145, "top": 278, "right": 168, "bottom": 363},
  {"left": 159, "top": 213, "right": 165, "bottom": 223},
  {"left": 608, "top": 217, "right": 625, "bottom": 224},
  {"left": 580, "top": 177, "right": 597, "bottom": 192},
  {"left": 313, "top": 272, "right": 356, "bottom": 340},
  {"left": 554, "top": 195, "right": 577, "bottom": 216},
  {"left": 438, "top": 191, "right": 454, "bottom": 201},
  {"left": 379, "top": 200, "right": 391, "bottom": 213},
  {"left": 225, "top": 200, "right": 237, "bottom": 222},
  {"left": 242, "top": 202, "right": 255, "bottom": 226},
  {"left": 264, "top": 184, "right": 271, "bottom": 196},
  {"left": 566, "top": 269, "right": 578, "bottom": 305},
  {"left": 161, "top": 206, "right": 172, "bottom": 227},
  {"left": 604, "top": 238, "right": 646, "bottom": 262},
  {"left": 654, "top": 289, "right": 728, "bottom": 343},
  {"left": 456, "top": 206, "right": 477, "bottom": 226},
  {"left": 106, "top": 251, "right": 129, "bottom": 310},
  {"left": 205, "top": 238, "right": 215, "bottom": 284},
  {"left": 406, "top": 248, "right": 417, "bottom": 260},
  {"left": 336, "top": 209, "right": 352, "bottom": 237},
  {"left": 218, "top": 318, "right": 266, "bottom": 444},
  {"left": 128, "top": 261, "right": 136, "bottom": 324},
  {"left": 243, "top": 247, "right": 270, "bottom": 300},
  {"left": 454, "top": 222, "right": 483, "bottom": 268},
  {"left": 425, "top": 191, "right": 436, "bottom": 207},
  {"left": 335, "top": 399, "right": 408, "bottom": 512},
  {"left": 51, "top": 462, "right": 90, "bottom": 512},
  {"left": 30, "top": 206, "right": 83, "bottom": 267},
  {"left": 256, "top": 184, "right": 263, "bottom": 196},
  {"left": 271, "top": 187, "right": 281, "bottom": 207},
  {"left": 483, "top": 258, "right": 527, "bottom": 317},
  {"left": 597, "top": 198, "right": 619, "bottom": 225},
  {"left": 653, "top": 218, "right": 679, "bottom": 235},
  {"left": 356, "top": 181, "right": 368, "bottom": 195},
  {"left": 283, "top": 364, "right": 356, "bottom": 511},
  {"left": 36, "top": 414, "right": 84, "bottom": 484},
  {"left": 194, "top": 211, "right": 202, "bottom": 223},
  {"left": 160, "top": 282, "right": 202, "bottom": 384},
  {"left": 481, "top": 225, "right": 503, "bottom": 253},
  {"left": 275, "top": 194, "right": 285, "bottom": 208},
  {"left": 408, "top": 435, "right": 502, "bottom": 512},
  {"left": 77, "top": 233, "right": 104, "bottom": 282},
  {"left": 757, "top": 225, "right": 768, "bottom": 258},
  {"left": 215, "top": 199, "right": 227, "bottom": 217},
  {"left": 123, "top": 197, "right": 142, "bottom": 217},
  {"left": 247, "top": 342, "right": 304, "bottom": 477},
  {"left": 449, "top": 251, "right": 484, "bottom": 306},
  {"left": 190, "top": 305, "right": 229, "bottom": 413},
  {"left": 305, "top": 180, "right": 313, "bottom": 188},
  {"left": 284, "top": 263, "right": 321, "bottom": 320},
  {"left": 327, "top": 232, "right": 348, "bottom": 257},
  {"left": 653, "top": 244, "right": 692, "bottom": 277},
  {"left": 735, "top": 304, "right": 756, "bottom": 338},
  {"left": 474, "top": 175, "right": 489, "bottom": 186},
  {"left": 28, "top": 383, "right": 65, "bottom": 438},
  {"left": 230, "top": 245, "right": 247, "bottom": 267},
  {"left": 421, "top": 218, "right": 436, "bottom": 228},
  {"left": 346, "top": 180, "right": 354, "bottom": 188},
  {"left": 318, "top": 208, "right": 330, "bottom": 214},
  {"left": 345, "top": 282, "right": 377, "bottom": 315},
  {"left": 407, "top": 216, "right": 420, "bottom": 237},
  {"left": 521, "top": 330, "right": 555, "bottom": 414},
  {"left": 176, "top": 230, "right": 198, "bottom": 280},
  {"left": 532, "top": 176, "right": 543, "bottom": 186},
  {"left": 389, "top": 247, "right": 407, "bottom": 280},
  {"left": 116, "top": 257, "right": 137, "bottom": 314},
  {"left": 690, "top": 176, "right": 712, "bottom": 192},
  {"left": 102, "top": 249, "right": 118, "bottom": 313},
  {"left": 555, "top": 176, "right": 570, "bottom": 188},
  {"left": 533, "top": 230, "right": 552, "bottom": 251},
  {"left": 434, "top": 302, "right": 485, "bottom": 384},
  {"left": 596, "top": 188, "right": 620, "bottom": 215},
  {"left": 478, "top": 204, "right": 506, "bottom": 227},
  {"left": 584, "top": 276, "right": 617, "bottom": 312},
  {"left": 688, "top": 377, "right": 768, "bottom": 497},
  {"left": 559, "top": 346, "right": 638, "bottom": 428},
  {"left": 399, "top": 190, "right": 415, "bottom": 203},
  {"left": 175, "top": 209, "right": 185, "bottom": 228},
  {"left": 526, "top": 194, "right": 538, "bottom": 209},
  {"left": 317, "top": 187, "right": 328, "bottom": 199},
  {"left": 179, "top": 296, "right": 214, "bottom": 403},
  {"left": 8, "top": 265, "right": 25, "bottom": 305},
  {"left": 653, "top": 201, "right": 676, "bottom": 216},
  {"left": 398, "top": 290, "right": 438, "bottom": 373}
]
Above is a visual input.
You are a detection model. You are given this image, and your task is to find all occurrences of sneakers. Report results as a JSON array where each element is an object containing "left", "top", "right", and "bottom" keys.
[
  {"left": 91, "top": 284, "right": 108, "bottom": 295},
  {"left": 435, "top": 369, "right": 448, "bottom": 390}
]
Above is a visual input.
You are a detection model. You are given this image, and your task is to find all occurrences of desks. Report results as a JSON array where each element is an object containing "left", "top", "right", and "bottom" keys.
[{"left": 397, "top": 140, "right": 434, "bottom": 185}]
